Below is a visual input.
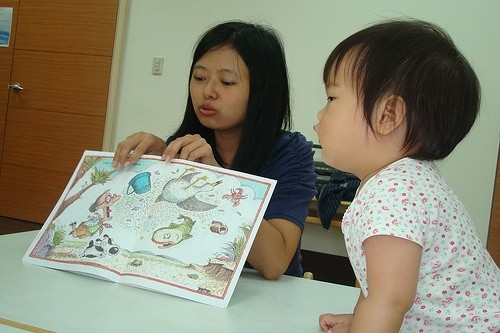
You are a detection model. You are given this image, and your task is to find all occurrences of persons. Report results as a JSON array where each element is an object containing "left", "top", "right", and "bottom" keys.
[
  {"left": 113, "top": 22, "right": 312, "bottom": 280},
  {"left": 313, "top": 21, "right": 500, "bottom": 333}
]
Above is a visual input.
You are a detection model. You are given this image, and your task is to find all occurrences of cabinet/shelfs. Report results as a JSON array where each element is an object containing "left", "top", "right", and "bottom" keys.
[{"left": 0, "top": 1, "right": 128, "bottom": 225}]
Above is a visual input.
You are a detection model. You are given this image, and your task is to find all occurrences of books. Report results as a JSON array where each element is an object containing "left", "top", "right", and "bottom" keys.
[{"left": 21, "top": 140, "right": 278, "bottom": 309}]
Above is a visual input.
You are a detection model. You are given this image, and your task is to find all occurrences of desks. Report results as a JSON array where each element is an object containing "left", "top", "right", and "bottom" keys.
[{"left": 0, "top": 228, "right": 361, "bottom": 333}]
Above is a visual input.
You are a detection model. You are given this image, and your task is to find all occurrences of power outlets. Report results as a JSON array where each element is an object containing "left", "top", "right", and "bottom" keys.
[{"left": 151, "top": 56, "right": 163, "bottom": 75}]
